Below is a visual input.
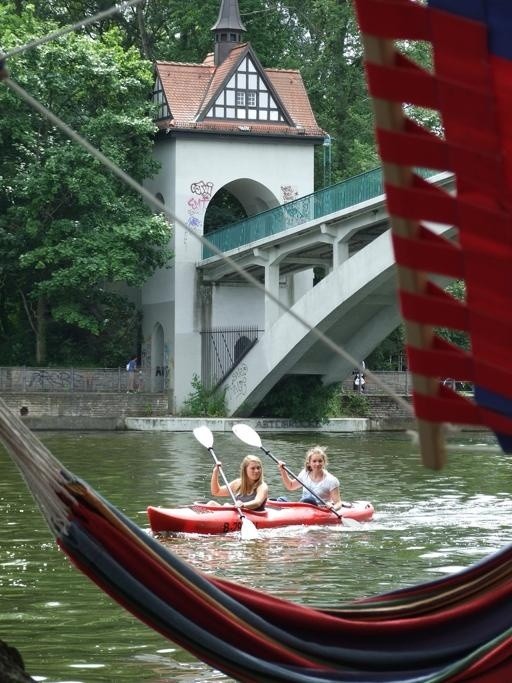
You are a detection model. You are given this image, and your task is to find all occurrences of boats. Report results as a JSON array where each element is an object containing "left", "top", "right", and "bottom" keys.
[{"left": 147, "top": 498, "right": 375, "bottom": 536}]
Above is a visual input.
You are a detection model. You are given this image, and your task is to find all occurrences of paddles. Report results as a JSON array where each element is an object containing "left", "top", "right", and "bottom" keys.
[
  {"left": 232, "top": 424, "right": 361, "bottom": 527},
  {"left": 193, "top": 426, "right": 262, "bottom": 540}
]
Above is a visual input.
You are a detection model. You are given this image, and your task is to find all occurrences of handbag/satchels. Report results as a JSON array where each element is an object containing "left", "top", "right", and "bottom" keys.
[{"left": 126, "top": 363, "right": 130, "bottom": 371}]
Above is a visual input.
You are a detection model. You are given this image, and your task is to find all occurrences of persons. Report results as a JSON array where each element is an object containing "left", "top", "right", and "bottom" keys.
[
  {"left": 206, "top": 454, "right": 268, "bottom": 512},
  {"left": 275, "top": 444, "right": 342, "bottom": 510},
  {"left": 127, "top": 356, "right": 140, "bottom": 393}
]
